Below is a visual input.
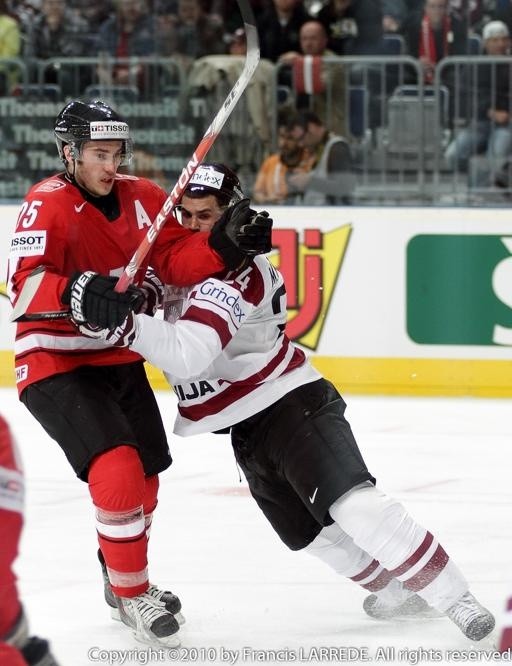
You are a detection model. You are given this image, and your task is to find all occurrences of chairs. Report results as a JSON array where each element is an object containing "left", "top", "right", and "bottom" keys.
[
  {"left": 246, "top": 0, "right": 512, "bottom": 210},
  {"left": 2, "top": 0, "right": 248, "bottom": 201}
]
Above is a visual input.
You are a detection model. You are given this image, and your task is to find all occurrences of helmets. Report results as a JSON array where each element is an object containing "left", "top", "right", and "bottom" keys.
[
  {"left": 184, "top": 163, "right": 245, "bottom": 209},
  {"left": 53, "top": 100, "right": 134, "bottom": 167}
]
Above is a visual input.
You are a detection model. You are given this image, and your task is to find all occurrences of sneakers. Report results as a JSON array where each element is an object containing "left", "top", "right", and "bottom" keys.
[
  {"left": 446, "top": 591, "right": 495, "bottom": 641},
  {"left": 363, "top": 593, "right": 429, "bottom": 618},
  {"left": 114, "top": 591, "right": 180, "bottom": 637},
  {"left": 102, "top": 578, "right": 181, "bottom": 613}
]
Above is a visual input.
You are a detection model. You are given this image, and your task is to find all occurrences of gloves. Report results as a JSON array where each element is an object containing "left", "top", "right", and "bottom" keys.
[
  {"left": 62, "top": 271, "right": 143, "bottom": 327},
  {"left": 214, "top": 198, "right": 274, "bottom": 262},
  {"left": 70, "top": 311, "right": 136, "bottom": 349}
]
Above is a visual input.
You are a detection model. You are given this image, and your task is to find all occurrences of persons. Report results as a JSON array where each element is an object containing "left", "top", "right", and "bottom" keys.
[
  {"left": 71, "top": 164, "right": 496, "bottom": 642},
  {"left": 2, "top": 0, "right": 510, "bottom": 206},
  {"left": 0, "top": 417, "right": 57, "bottom": 665},
  {"left": 8, "top": 97, "right": 271, "bottom": 646}
]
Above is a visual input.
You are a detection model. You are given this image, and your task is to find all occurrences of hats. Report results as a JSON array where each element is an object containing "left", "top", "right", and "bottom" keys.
[{"left": 478, "top": 20, "right": 507, "bottom": 52}]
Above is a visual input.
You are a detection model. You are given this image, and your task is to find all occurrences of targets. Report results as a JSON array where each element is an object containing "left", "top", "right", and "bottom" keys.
[{"left": 114, "top": 2, "right": 259, "bottom": 295}]
